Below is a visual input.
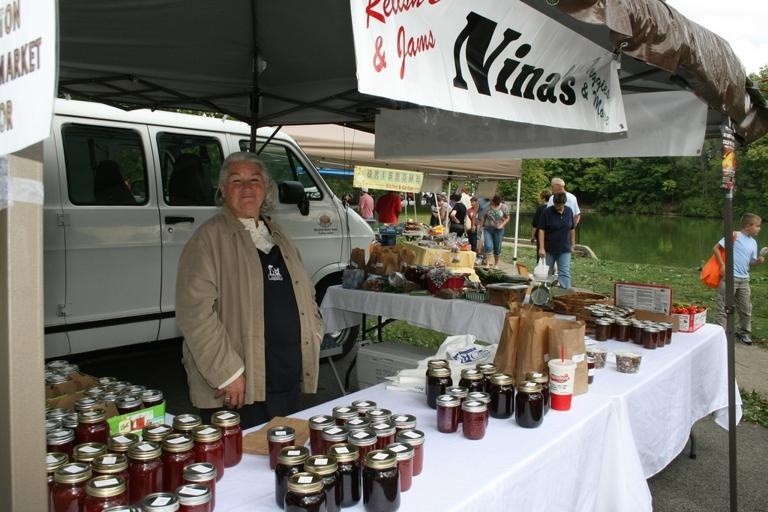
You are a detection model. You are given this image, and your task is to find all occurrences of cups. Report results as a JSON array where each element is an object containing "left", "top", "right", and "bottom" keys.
[{"left": 548, "top": 358, "right": 578, "bottom": 411}]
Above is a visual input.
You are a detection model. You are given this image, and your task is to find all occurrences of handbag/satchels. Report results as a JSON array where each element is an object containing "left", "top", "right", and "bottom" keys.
[
  {"left": 463, "top": 214, "right": 472, "bottom": 231},
  {"left": 449, "top": 223, "right": 465, "bottom": 234}
]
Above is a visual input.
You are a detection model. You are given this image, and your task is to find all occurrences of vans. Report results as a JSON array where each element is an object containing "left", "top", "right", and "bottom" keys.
[{"left": 44, "top": 95, "right": 376, "bottom": 364}]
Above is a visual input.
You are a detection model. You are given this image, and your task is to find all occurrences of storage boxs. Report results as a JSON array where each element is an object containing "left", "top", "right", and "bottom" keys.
[
  {"left": 425, "top": 275, "right": 465, "bottom": 294},
  {"left": 484, "top": 283, "right": 530, "bottom": 309},
  {"left": 354, "top": 340, "right": 437, "bottom": 390},
  {"left": 44, "top": 371, "right": 166, "bottom": 440},
  {"left": 632, "top": 306, "right": 707, "bottom": 334}
]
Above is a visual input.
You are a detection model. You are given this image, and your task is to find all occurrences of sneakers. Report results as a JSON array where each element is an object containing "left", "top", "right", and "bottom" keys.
[{"left": 738, "top": 332, "right": 754, "bottom": 345}]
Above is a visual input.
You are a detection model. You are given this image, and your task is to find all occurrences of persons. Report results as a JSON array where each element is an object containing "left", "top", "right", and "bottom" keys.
[
  {"left": 359, "top": 188, "right": 375, "bottom": 219},
  {"left": 547, "top": 178, "right": 581, "bottom": 286},
  {"left": 432, "top": 186, "right": 511, "bottom": 269},
  {"left": 530, "top": 191, "right": 550, "bottom": 262},
  {"left": 175, "top": 152, "right": 326, "bottom": 428},
  {"left": 537, "top": 192, "right": 576, "bottom": 289},
  {"left": 712, "top": 213, "right": 764, "bottom": 346},
  {"left": 376, "top": 191, "right": 402, "bottom": 245}
]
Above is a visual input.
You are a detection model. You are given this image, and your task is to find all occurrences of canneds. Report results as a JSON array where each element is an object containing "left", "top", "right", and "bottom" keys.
[
  {"left": 585, "top": 303, "right": 673, "bottom": 349},
  {"left": 45, "top": 360, "right": 242, "bottom": 512},
  {"left": 266, "top": 400, "right": 425, "bottom": 512},
  {"left": 426, "top": 360, "right": 549, "bottom": 440},
  {"left": 587, "top": 357, "right": 596, "bottom": 384}
]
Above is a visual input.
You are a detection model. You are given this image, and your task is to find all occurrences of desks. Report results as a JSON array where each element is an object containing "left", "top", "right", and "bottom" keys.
[
  {"left": 400, "top": 240, "right": 477, "bottom": 270},
  {"left": 179, "top": 359, "right": 654, "bottom": 512},
  {"left": 316, "top": 278, "right": 727, "bottom": 465}
]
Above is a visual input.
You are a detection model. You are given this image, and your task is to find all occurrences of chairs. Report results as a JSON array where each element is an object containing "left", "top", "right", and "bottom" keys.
[
  {"left": 166, "top": 152, "right": 215, "bottom": 206},
  {"left": 92, "top": 159, "right": 139, "bottom": 205}
]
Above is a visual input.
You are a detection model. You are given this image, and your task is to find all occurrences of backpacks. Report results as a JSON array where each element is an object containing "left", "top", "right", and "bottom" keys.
[{"left": 699, "top": 231, "right": 737, "bottom": 289}]
